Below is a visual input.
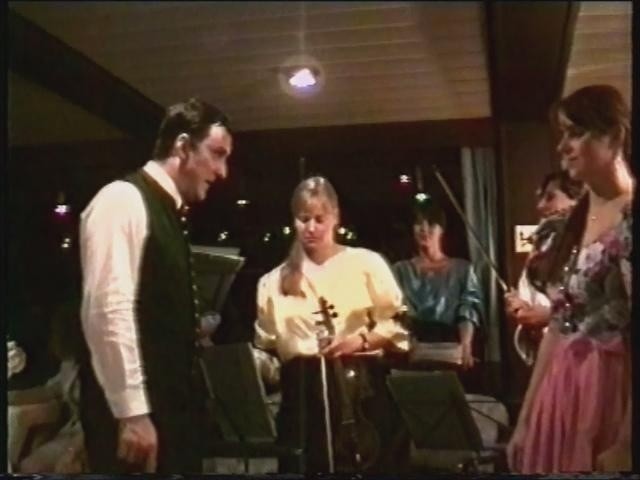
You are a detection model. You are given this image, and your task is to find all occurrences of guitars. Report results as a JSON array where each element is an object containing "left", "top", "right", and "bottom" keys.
[{"left": 311, "top": 296, "right": 381, "bottom": 474}]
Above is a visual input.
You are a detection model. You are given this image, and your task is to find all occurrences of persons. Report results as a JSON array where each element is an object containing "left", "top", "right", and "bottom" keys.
[
  {"left": 390, "top": 203, "right": 481, "bottom": 395},
  {"left": 503, "top": 84, "right": 636, "bottom": 478},
  {"left": 252, "top": 174, "right": 413, "bottom": 480},
  {"left": 77, "top": 98, "right": 236, "bottom": 479},
  {"left": 502, "top": 170, "right": 585, "bottom": 368}
]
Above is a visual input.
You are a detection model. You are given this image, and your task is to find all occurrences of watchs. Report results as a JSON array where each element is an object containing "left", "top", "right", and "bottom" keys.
[{"left": 360, "top": 332, "right": 370, "bottom": 354}]
